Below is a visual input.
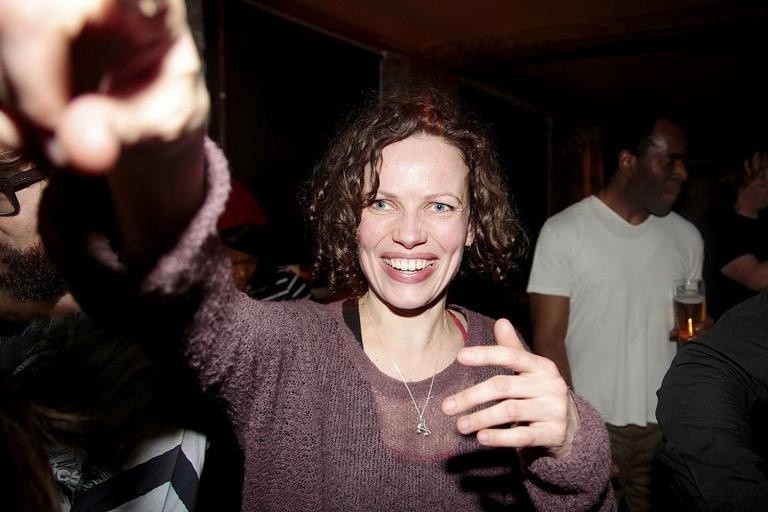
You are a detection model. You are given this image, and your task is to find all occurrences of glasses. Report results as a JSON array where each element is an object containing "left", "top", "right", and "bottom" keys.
[{"left": 0, "top": 169, "right": 48, "bottom": 219}]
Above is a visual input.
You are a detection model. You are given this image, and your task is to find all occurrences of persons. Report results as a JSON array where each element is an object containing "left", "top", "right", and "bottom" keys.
[
  {"left": 708, "top": 136, "right": 767, "bottom": 297},
  {"left": 1, "top": 0, "right": 622, "bottom": 511},
  {"left": 2, "top": 103, "right": 357, "bottom": 509},
  {"left": 522, "top": 103, "right": 709, "bottom": 510},
  {"left": 646, "top": 291, "right": 767, "bottom": 510}
]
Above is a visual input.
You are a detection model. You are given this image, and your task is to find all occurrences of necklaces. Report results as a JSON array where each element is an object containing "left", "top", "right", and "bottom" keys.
[{"left": 372, "top": 339, "right": 448, "bottom": 441}]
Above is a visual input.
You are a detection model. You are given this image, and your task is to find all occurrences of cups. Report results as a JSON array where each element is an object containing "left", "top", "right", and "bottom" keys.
[{"left": 671, "top": 277, "right": 708, "bottom": 346}]
